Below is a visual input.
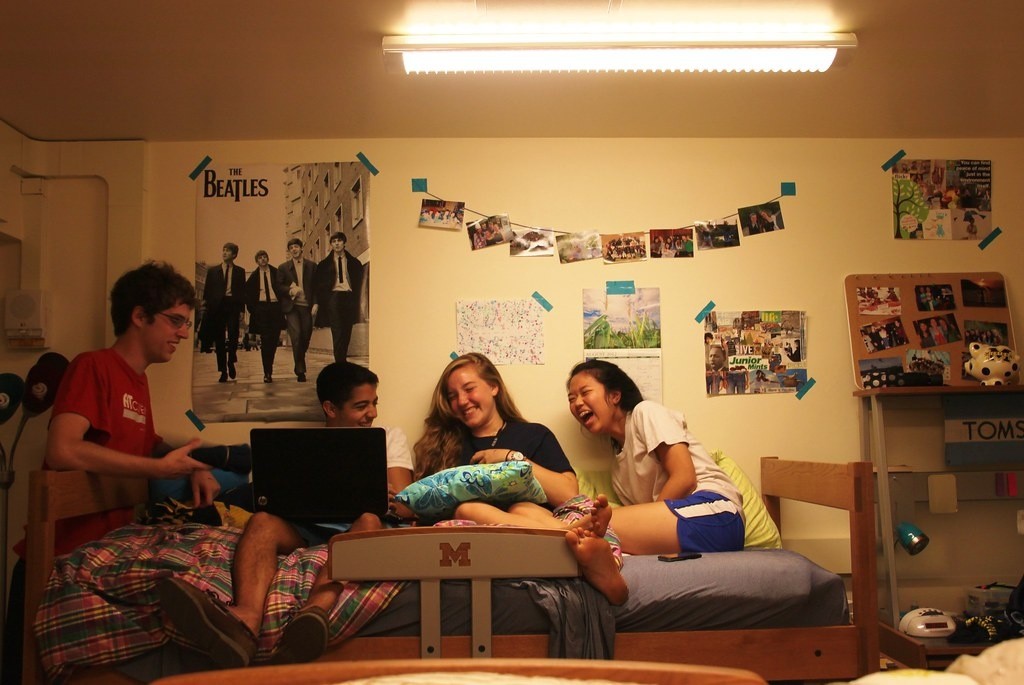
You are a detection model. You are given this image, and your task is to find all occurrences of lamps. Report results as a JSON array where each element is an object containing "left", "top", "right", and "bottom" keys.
[
  {"left": 894, "top": 521, "right": 940, "bottom": 619},
  {"left": 382, "top": 32, "right": 859, "bottom": 73}
]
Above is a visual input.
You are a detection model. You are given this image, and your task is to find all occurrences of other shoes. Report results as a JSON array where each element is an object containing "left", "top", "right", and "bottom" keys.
[
  {"left": 264, "top": 373, "right": 272, "bottom": 382},
  {"left": 298, "top": 374, "right": 306, "bottom": 382},
  {"left": 227, "top": 361, "right": 236, "bottom": 379},
  {"left": 219, "top": 373, "right": 228, "bottom": 382}
]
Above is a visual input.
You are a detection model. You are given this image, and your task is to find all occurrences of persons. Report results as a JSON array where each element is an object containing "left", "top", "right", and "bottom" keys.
[
  {"left": 742, "top": 210, "right": 780, "bottom": 236},
  {"left": 414, "top": 353, "right": 629, "bottom": 606},
  {"left": 651, "top": 235, "right": 693, "bottom": 258},
  {"left": 606, "top": 237, "right": 646, "bottom": 262},
  {"left": 1, "top": 259, "right": 221, "bottom": 685},
  {"left": 705, "top": 329, "right": 800, "bottom": 394},
  {"left": 701, "top": 228, "right": 713, "bottom": 248},
  {"left": 892, "top": 161, "right": 989, "bottom": 240},
  {"left": 474, "top": 222, "right": 506, "bottom": 249},
  {"left": 197, "top": 231, "right": 364, "bottom": 383},
  {"left": 567, "top": 361, "right": 746, "bottom": 556},
  {"left": 419, "top": 206, "right": 464, "bottom": 224},
  {"left": 160, "top": 363, "right": 414, "bottom": 669},
  {"left": 857, "top": 287, "right": 1002, "bottom": 372}
]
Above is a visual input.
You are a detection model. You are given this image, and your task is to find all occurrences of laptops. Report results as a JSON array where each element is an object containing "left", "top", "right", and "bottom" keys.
[{"left": 250, "top": 427, "right": 389, "bottom": 523}]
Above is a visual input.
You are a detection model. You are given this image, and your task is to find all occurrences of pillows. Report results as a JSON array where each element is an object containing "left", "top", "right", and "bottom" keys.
[{"left": 394, "top": 461, "right": 548, "bottom": 524}]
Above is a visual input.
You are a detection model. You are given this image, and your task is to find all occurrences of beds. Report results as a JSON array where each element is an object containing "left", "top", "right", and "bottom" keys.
[{"left": 21, "top": 454, "right": 880, "bottom": 685}]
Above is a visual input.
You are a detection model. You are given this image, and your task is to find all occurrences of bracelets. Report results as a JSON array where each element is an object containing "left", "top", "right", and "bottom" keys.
[{"left": 505, "top": 450, "right": 512, "bottom": 461}]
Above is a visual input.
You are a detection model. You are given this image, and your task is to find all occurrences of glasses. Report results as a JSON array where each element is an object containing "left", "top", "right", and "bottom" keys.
[{"left": 157, "top": 311, "right": 192, "bottom": 330}]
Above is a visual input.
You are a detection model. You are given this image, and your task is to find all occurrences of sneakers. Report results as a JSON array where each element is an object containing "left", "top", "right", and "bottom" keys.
[
  {"left": 159, "top": 576, "right": 261, "bottom": 669},
  {"left": 271, "top": 608, "right": 329, "bottom": 664}
]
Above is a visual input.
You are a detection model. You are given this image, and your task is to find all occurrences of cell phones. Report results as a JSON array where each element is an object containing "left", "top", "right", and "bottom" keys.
[{"left": 658, "top": 553, "right": 702, "bottom": 562}]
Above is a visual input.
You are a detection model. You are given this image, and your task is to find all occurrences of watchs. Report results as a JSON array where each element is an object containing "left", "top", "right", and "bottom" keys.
[{"left": 511, "top": 451, "right": 524, "bottom": 461}]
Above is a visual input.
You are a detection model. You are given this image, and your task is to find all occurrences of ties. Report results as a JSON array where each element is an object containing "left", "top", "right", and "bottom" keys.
[
  {"left": 264, "top": 273, "right": 272, "bottom": 303},
  {"left": 224, "top": 265, "right": 230, "bottom": 294},
  {"left": 338, "top": 256, "right": 344, "bottom": 283}
]
